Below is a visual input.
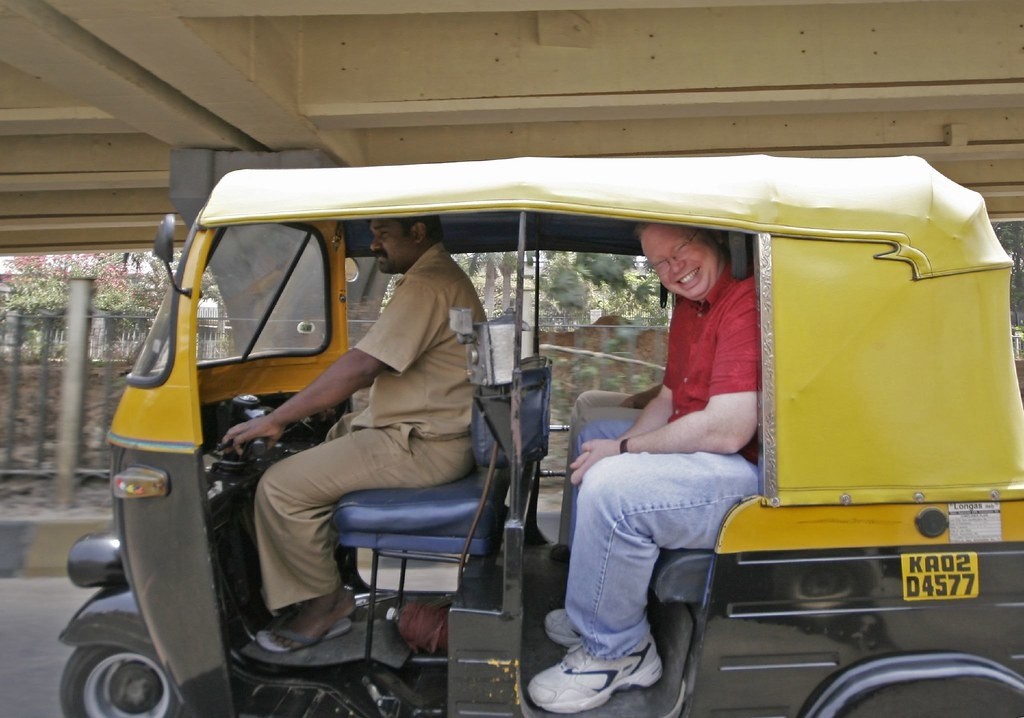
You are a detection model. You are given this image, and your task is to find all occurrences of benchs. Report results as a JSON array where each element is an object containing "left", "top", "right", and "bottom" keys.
[{"left": 650, "top": 546, "right": 712, "bottom": 605}]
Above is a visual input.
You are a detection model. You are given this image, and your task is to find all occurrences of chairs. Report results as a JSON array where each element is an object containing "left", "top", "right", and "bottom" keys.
[{"left": 334, "top": 354, "right": 555, "bottom": 670}]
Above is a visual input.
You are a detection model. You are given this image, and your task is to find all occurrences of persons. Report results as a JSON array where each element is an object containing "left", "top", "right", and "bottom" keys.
[
  {"left": 527, "top": 219, "right": 764, "bottom": 713},
  {"left": 221, "top": 215, "right": 488, "bottom": 653}
]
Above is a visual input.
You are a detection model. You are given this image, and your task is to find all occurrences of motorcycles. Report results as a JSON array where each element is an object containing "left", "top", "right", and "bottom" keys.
[{"left": 55, "top": 150, "right": 1024, "bottom": 717}]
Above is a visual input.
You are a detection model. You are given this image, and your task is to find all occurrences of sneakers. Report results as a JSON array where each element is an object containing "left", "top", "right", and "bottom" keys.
[
  {"left": 541, "top": 604, "right": 577, "bottom": 650},
  {"left": 527, "top": 645, "right": 663, "bottom": 716}
]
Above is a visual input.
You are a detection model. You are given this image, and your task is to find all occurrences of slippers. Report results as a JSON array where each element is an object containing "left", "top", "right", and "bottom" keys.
[{"left": 255, "top": 615, "right": 354, "bottom": 654}]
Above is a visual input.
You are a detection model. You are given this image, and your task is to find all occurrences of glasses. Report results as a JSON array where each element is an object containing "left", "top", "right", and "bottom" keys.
[{"left": 652, "top": 229, "right": 701, "bottom": 277}]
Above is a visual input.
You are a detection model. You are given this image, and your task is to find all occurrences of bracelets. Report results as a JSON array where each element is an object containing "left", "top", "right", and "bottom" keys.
[{"left": 619, "top": 436, "right": 628, "bottom": 453}]
